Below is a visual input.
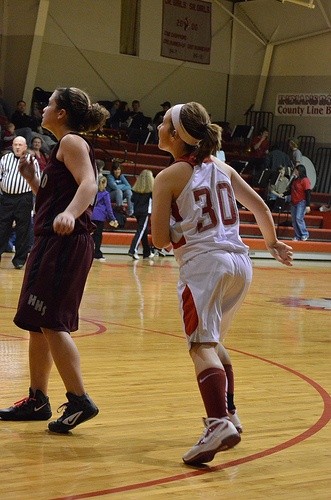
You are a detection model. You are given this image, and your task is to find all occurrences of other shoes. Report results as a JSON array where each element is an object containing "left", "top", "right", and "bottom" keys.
[
  {"left": 95, "top": 257, "right": 104, "bottom": 259},
  {"left": 292, "top": 237, "right": 299, "bottom": 241},
  {"left": 128, "top": 210, "right": 134, "bottom": 216},
  {"left": 16, "top": 265, "right": 22, "bottom": 268},
  {"left": 148, "top": 253, "right": 154, "bottom": 258},
  {"left": 301, "top": 236, "right": 308, "bottom": 240},
  {"left": 129, "top": 252, "right": 139, "bottom": 259}
]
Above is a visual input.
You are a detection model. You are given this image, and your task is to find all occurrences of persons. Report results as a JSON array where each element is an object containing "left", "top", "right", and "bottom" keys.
[
  {"left": 250, "top": 128, "right": 302, "bottom": 226},
  {"left": 0, "top": 88, "right": 110, "bottom": 433},
  {"left": 0, "top": 136, "right": 41, "bottom": 269},
  {"left": 90, "top": 177, "right": 118, "bottom": 261},
  {"left": 150, "top": 101, "right": 294, "bottom": 464},
  {"left": 91, "top": 159, "right": 135, "bottom": 218},
  {"left": 0, "top": 100, "right": 57, "bottom": 254},
  {"left": 128, "top": 170, "right": 155, "bottom": 260},
  {"left": 99, "top": 100, "right": 172, "bottom": 144},
  {"left": 280, "top": 164, "right": 311, "bottom": 241}
]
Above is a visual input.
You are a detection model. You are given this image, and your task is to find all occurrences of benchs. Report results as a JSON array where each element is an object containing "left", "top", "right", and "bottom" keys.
[{"left": 86, "top": 128, "right": 331, "bottom": 242}]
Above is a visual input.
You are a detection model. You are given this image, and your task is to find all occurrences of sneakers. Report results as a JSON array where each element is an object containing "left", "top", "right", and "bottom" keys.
[
  {"left": 229, "top": 411, "right": 242, "bottom": 433},
  {"left": 48, "top": 393, "right": 99, "bottom": 433},
  {"left": 181, "top": 417, "right": 241, "bottom": 463},
  {"left": 0, "top": 388, "right": 52, "bottom": 420}
]
